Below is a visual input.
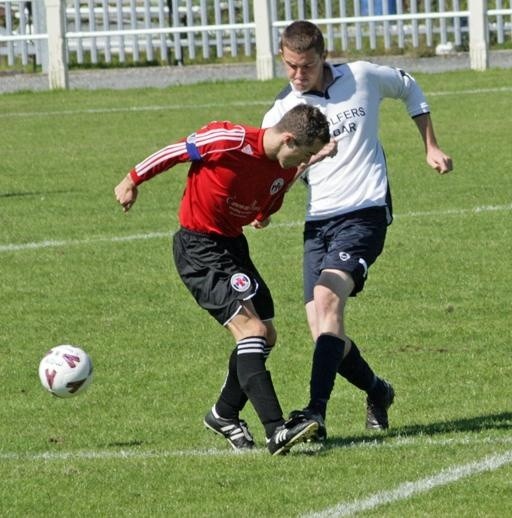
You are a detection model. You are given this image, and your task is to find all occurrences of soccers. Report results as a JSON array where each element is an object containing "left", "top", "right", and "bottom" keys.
[{"left": 38, "top": 344, "right": 93, "bottom": 398}]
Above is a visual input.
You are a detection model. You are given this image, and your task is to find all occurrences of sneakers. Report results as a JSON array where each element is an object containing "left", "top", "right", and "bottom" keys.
[
  {"left": 265, "top": 410, "right": 326, "bottom": 457},
  {"left": 366, "top": 377, "right": 395, "bottom": 431},
  {"left": 203, "top": 404, "right": 256, "bottom": 451}
]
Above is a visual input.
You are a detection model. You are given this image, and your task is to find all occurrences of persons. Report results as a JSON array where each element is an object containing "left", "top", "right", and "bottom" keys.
[
  {"left": 114, "top": 103, "right": 330, "bottom": 457},
  {"left": 260, "top": 19, "right": 453, "bottom": 445}
]
